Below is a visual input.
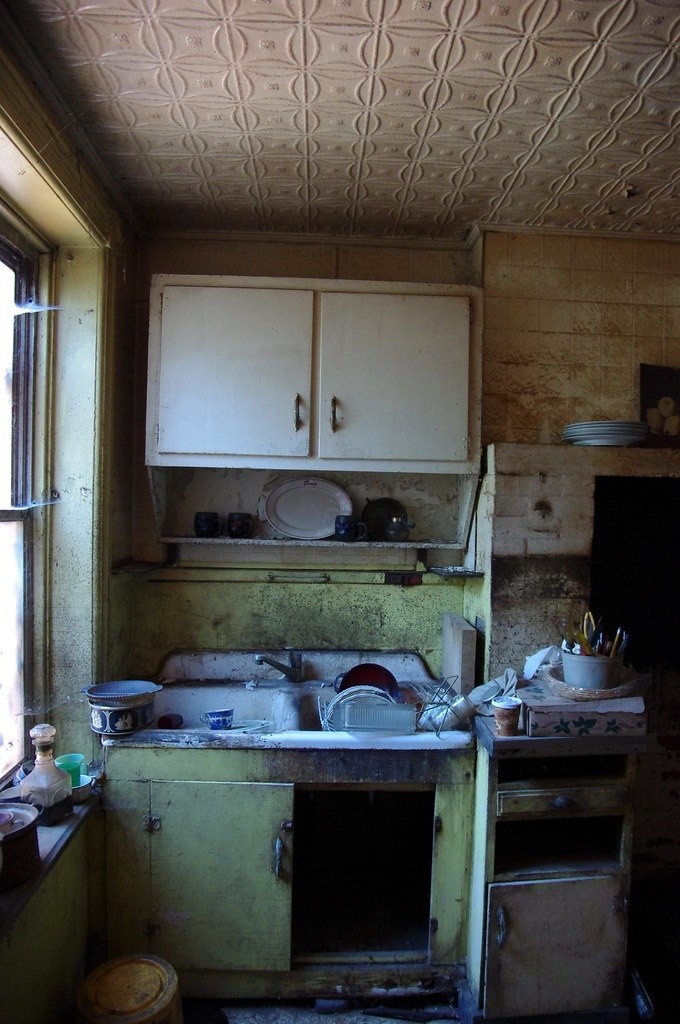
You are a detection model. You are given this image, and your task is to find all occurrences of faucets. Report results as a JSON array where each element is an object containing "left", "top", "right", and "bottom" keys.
[{"left": 254, "top": 646, "right": 305, "bottom": 687}]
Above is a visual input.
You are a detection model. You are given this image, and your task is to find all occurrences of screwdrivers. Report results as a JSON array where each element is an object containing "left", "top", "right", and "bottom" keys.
[
  {"left": 574, "top": 630, "right": 594, "bottom": 657},
  {"left": 561, "top": 622, "right": 575, "bottom": 655}
]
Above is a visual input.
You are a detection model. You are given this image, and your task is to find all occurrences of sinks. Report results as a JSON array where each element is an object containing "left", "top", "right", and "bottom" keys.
[
  {"left": 280, "top": 678, "right": 475, "bottom": 751},
  {"left": 145, "top": 676, "right": 302, "bottom": 750}
]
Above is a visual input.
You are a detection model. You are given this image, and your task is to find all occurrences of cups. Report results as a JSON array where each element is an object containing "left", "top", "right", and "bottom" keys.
[
  {"left": 199, "top": 709, "right": 233, "bottom": 730},
  {"left": 194, "top": 512, "right": 222, "bottom": 538},
  {"left": 58, "top": 761, "right": 80, "bottom": 787},
  {"left": 428, "top": 693, "right": 476, "bottom": 730},
  {"left": 55, "top": 753, "right": 88, "bottom": 776},
  {"left": 228, "top": 513, "right": 253, "bottom": 538},
  {"left": 561, "top": 655, "right": 623, "bottom": 689},
  {"left": 72, "top": 775, "right": 97, "bottom": 802},
  {"left": 335, "top": 515, "right": 367, "bottom": 541},
  {"left": 158, "top": 714, "right": 184, "bottom": 729},
  {"left": 12, "top": 763, "right": 35, "bottom": 785}
]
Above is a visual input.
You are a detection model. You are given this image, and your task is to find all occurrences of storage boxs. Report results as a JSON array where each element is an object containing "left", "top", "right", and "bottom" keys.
[{"left": 517, "top": 687, "right": 647, "bottom": 738}]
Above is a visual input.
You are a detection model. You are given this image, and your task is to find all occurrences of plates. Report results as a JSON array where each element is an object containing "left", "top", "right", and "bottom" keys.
[
  {"left": 264, "top": 477, "right": 352, "bottom": 540},
  {"left": 563, "top": 420, "right": 648, "bottom": 446}
]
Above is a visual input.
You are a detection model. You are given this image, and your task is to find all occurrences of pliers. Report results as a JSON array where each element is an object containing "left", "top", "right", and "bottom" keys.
[{"left": 584, "top": 612, "right": 596, "bottom": 642}]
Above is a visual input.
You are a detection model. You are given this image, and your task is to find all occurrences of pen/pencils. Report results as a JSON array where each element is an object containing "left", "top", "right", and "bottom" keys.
[
  {"left": 609, "top": 627, "right": 622, "bottom": 657},
  {"left": 618, "top": 635, "right": 629, "bottom": 656}
]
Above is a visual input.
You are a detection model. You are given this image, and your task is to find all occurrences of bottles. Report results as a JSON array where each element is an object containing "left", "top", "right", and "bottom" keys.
[
  {"left": 491, "top": 697, "right": 522, "bottom": 736},
  {"left": 19, "top": 724, "right": 74, "bottom": 826}
]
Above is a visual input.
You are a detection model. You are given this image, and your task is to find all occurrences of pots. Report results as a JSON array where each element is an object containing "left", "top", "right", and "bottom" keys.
[
  {"left": 81, "top": 680, "right": 163, "bottom": 734},
  {"left": 334, "top": 663, "right": 399, "bottom": 699},
  {"left": 0, "top": 801, "right": 45, "bottom": 889}
]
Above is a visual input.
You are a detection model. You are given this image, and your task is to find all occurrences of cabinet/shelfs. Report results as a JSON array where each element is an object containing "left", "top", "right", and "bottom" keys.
[
  {"left": 144, "top": 273, "right": 485, "bottom": 550},
  {"left": 101, "top": 747, "right": 478, "bottom": 1002},
  {"left": 466, "top": 715, "right": 648, "bottom": 1018}
]
